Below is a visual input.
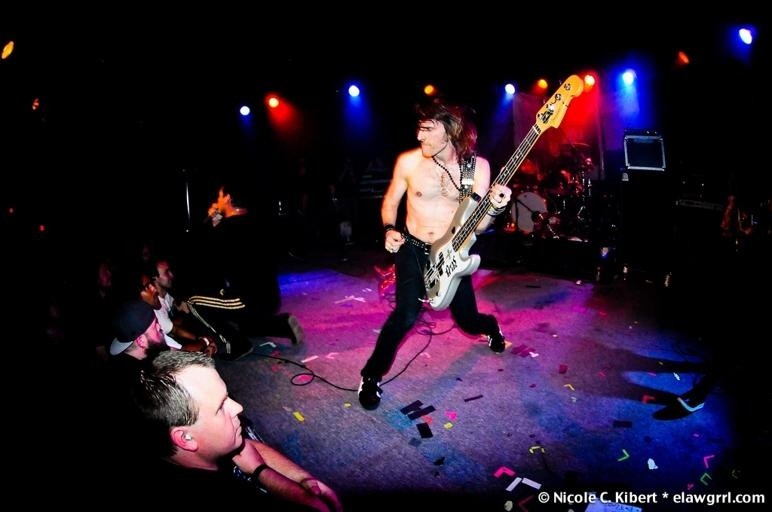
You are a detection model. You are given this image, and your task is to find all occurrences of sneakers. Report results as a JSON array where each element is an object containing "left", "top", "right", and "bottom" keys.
[
  {"left": 219, "top": 343, "right": 253, "bottom": 362},
  {"left": 285, "top": 315, "right": 304, "bottom": 344},
  {"left": 358, "top": 377, "right": 382, "bottom": 411},
  {"left": 489, "top": 315, "right": 505, "bottom": 352}
]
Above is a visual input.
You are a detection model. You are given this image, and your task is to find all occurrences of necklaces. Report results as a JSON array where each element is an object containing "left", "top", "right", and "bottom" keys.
[{"left": 431, "top": 156, "right": 466, "bottom": 191}]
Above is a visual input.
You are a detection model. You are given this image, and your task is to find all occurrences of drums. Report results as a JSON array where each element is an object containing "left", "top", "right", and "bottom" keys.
[{"left": 511, "top": 192, "right": 557, "bottom": 234}]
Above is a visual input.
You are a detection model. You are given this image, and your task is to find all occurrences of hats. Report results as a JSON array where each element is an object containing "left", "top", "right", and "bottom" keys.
[{"left": 110, "top": 301, "right": 156, "bottom": 355}]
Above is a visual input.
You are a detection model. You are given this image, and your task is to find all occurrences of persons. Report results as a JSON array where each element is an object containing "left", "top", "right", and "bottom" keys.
[
  {"left": 99, "top": 174, "right": 344, "bottom": 512},
  {"left": 511, "top": 175, "right": 560, "bottom": 238},
  {"left": 357, "top": 98, "right": 513, "bottom": 410},
  {"left": 677, "top": 188, "right": 772, "bottom": 414}
]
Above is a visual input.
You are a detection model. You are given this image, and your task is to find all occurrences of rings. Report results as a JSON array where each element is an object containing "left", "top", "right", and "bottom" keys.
[
  {"left": 499, "top": 193, "right": 504, "bottom": 198},
  {"left": 497, "top": 200, "right": 503, "bottom": 204}
]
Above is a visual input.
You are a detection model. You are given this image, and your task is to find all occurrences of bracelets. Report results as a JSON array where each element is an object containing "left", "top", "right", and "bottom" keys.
[{"left": 382, "top": 224, "right": 396, "bottom": 232}]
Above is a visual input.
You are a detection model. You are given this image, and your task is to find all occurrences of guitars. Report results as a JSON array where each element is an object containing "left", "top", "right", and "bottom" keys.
[{"left": 425, "top": 74, "right": 584, "bottom": 311}]
